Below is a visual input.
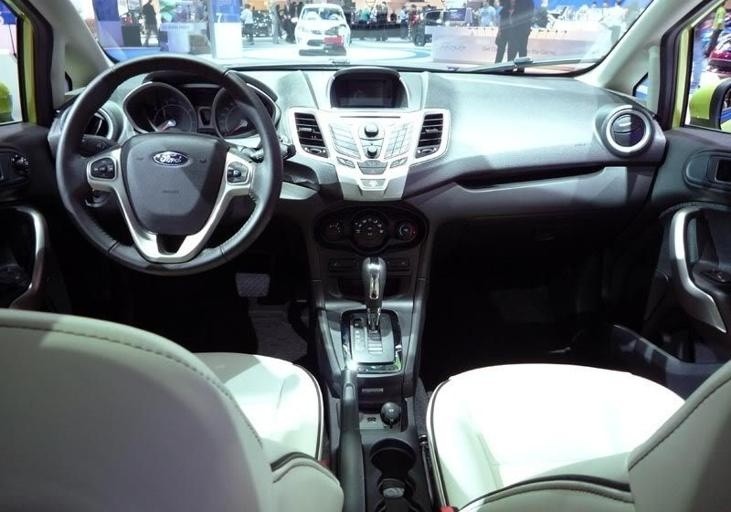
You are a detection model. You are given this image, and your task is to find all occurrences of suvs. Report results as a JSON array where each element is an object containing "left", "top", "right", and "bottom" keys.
[{"left": 408, "top": 7, "right": 474, "bottom": 46}]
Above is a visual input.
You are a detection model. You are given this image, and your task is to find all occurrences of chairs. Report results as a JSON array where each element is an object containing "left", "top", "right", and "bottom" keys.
[
  {"left": 0, "top": 300, "right": 344, "bottom": 512},
  {"left": 421, "top": 346, "right": 730, "bottom": 512}
]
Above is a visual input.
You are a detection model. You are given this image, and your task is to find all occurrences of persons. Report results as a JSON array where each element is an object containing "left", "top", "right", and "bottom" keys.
[
  {"left": 703, "top": 1, "right": 727, "bottom": 57},
  {"left": 142, "top": 0, "right": 160, "bottom": 46},
  {"left": 240, "top": 3, "right": 255, "bottom": 45}
]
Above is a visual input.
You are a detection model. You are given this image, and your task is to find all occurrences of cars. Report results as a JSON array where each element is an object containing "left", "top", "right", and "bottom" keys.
[
  {"left": 700, "top": 11, "right": 731, "bottom": 70},
  {"left": 243, "top": 13, "right": 269, "bottom": 37},
  {"left": 291, "top": 3, "right": 351, "bottom": 55}
]
